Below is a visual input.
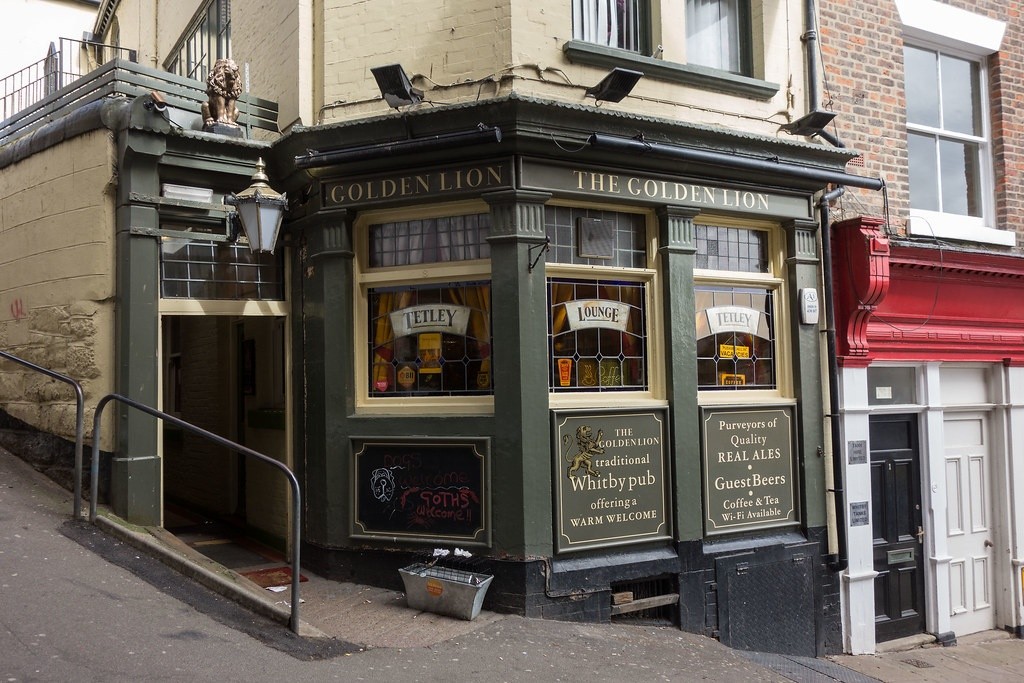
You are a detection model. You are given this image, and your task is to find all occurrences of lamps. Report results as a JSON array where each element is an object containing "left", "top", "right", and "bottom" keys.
[
  {"left": 369, "top": 63, "right": 433, "bottom": 112},
  {"left": 222, "top": 155, "right": 292, "bottom": 257},
  {"left": 584, "top": 65, "right": 644, "bottom": 106},
  {"left": 778, "top": 107, "right": 838, "bottom": 142}
]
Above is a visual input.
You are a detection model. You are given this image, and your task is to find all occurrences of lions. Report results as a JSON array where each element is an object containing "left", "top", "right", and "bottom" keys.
[
  {"left": 562, "top": 426, "right": 606, "bottom": 480},
  {"left": 201, "top": 58, "right": 242, "bottom": 125}
]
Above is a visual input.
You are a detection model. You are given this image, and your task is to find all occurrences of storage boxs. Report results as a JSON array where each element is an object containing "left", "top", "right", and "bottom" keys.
[{"left": 399, "top": 562, "right": 495, "bottom": 622}]
